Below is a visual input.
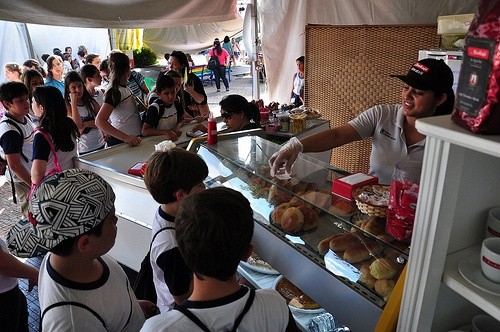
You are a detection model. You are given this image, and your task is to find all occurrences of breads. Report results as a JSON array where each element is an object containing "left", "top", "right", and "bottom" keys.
[
  {"left": 232, "top": 164, "right": 413, "bottom": 300},
  {"left": 275, "top": 278, "right": 322, "bottom": 309}
]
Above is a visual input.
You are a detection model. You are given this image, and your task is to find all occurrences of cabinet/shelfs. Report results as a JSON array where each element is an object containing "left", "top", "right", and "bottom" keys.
[
  {"left": 186, "top": 128, "right": 411, "bottom": 332},
  {"left": 395, "top": 116, "right": 500, "bottom": 332}
]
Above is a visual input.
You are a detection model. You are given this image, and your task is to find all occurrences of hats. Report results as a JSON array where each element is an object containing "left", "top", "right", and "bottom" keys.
[
  {"left": 6, "top": 169, "right": 115, "bottom": 259},
  {"left": 390, "top": 58, "right": 453, "bottom": 92},
  {"left": 164, "top": 50, "right": 189, "bottom": 68}
]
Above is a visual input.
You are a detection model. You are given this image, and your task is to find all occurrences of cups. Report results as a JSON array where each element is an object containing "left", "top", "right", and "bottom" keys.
[
  {"left": 484, "top": 207, "right": 500, "bottom": 237},
  {"left": 480, "top": 237, "right": 500, "bottom": 283},
  {"left": 259, "top": 104, "right": 306, "bottom": 134},
  {"left": 471, "top": 314, "right": 498, "bottom": 332}
]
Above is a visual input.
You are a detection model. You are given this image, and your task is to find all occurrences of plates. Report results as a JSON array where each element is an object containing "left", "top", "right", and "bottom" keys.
[
  {"left": 240, "top": 259, "right": 280, "bottom": 276},
  {"left": 289, "top": 108, "right": 323, "bottom": 119},
  {"left": 458, "top": 254, "right": 500, "bottom": 297},
  {"left": 272, "top": 274, "right": 325, "bottom": 314}
]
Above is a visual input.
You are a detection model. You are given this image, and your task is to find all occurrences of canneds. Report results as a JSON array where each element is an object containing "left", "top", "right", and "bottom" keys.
[{"left": 276, "top": 114, "right": 290, "bottom": 132}]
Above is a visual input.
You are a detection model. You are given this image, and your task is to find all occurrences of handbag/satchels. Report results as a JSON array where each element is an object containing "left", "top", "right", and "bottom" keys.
[{"left": 28, "top": 166, "right": 63, "bottom": 203}]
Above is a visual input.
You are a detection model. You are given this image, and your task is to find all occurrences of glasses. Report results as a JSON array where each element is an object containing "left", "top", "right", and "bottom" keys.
[{"left": 221, "top": 112, "right": 232, "bottom": 119}]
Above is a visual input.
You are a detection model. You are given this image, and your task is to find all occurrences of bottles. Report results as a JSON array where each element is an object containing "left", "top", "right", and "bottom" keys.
[{"left": 206, "top": 112, "right": 218, "bottom": 146}]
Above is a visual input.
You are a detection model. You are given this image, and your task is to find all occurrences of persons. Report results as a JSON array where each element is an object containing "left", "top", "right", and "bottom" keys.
[
  {"left": 0, "top": 35, "right": 296, "bottom": 332},
  {"left": 138, "top": 187, "right": 297, "bottom": 332},
  {"left": 291, "top": 56, "right": 304, "bottom": 107},
  {"left": 4, "top": 168, "right": 145, "bottom": 332},
  {"left": 267, "top": 58, "right": 455, "bottom": 185}
]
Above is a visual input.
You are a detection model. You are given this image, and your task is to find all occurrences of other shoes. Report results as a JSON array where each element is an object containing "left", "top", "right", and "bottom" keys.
[
  {"left": 217, "top": 89, "right": 220, "bottom": 92},
  {"left": 226, "top": 87, "right": 229, "bottom": 91}
]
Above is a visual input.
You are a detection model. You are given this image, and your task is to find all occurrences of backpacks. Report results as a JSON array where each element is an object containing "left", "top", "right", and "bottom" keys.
[{"left": 207, "top": 49, "right": 219, "bottom": 71}]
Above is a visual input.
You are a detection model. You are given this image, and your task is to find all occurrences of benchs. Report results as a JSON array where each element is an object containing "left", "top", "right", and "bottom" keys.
[{"left": 189, "top": 60, "right": 233, "bottom": 86}]
